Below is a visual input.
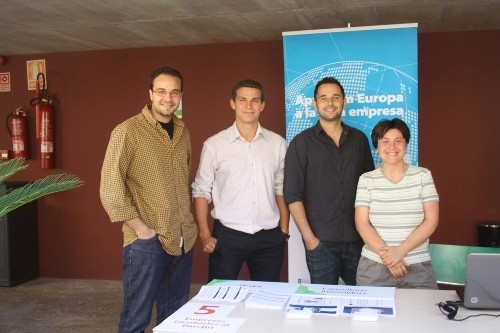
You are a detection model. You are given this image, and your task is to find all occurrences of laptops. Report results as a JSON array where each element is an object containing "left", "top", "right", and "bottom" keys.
[{"left": 456, "top": 252, "right": 500, "bottom": 310}]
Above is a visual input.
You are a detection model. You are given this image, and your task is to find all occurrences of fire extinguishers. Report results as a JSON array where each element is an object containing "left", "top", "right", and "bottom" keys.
[
  {"left": 10, "top": 105, "right": 30, "bottom": 161},
  {"left": 39, "top": 97, "right": 55, "bottom": 169}
]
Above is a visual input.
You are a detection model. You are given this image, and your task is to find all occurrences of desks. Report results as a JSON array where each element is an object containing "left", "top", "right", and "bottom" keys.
[{"left": 151, "top": 278, "right": 499, "bottom": 332}]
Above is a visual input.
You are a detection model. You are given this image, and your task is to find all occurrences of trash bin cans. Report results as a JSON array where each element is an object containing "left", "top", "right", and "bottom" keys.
[
  {"left": 477, "top": 223, "right": 500, "bottom": 248},
  {"left": 0, "top": 182, "right": 38, "bottom": 286}
]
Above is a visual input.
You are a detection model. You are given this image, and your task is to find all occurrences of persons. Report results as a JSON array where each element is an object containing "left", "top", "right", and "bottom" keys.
[
  {"left": 100, "top": 66, "right": 198, "bottom": 333},
  {"left": 283, "top": 76, "right": 377, "bottom": 285},
  {"left": 191, "top": 80, "right": 290, "bottom": 282},
  {"left": 354, "top": 118, "right": 441, "bottom": 290}
]
